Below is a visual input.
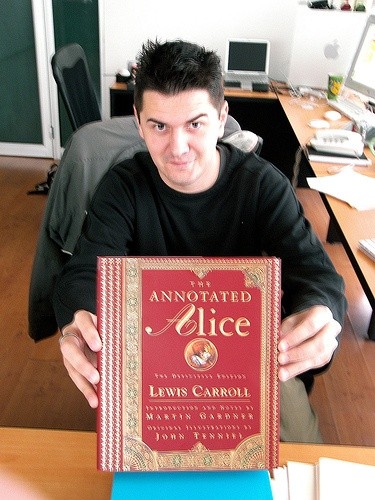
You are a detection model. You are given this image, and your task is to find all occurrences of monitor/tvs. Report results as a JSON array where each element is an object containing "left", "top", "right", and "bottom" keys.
[
  {"left": 223, "top": 38, "right": 270, "bottom": 76},
  {"left": 343, "top": 14, "right": 375, "bottom": 97}
]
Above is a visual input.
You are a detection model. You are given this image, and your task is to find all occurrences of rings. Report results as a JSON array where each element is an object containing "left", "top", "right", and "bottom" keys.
[{"left": 60, "top": 333, "right": 79, "bottom": 343}]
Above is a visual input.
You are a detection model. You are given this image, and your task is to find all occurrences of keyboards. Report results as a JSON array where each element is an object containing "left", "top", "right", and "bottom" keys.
[
  {"left": 326, "top": 98, "right": 375, "bottom": 128},
  {"left": 224, "top": 73, "right": 270, "bottom": 84}
]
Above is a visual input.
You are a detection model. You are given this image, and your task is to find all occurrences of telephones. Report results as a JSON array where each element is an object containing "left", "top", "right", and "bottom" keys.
[{"left": 310, "top": 129, "right": 364, "bottom": 158}]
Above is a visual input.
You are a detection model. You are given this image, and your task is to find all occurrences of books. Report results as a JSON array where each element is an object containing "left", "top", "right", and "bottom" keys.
[
  {"left": 306, "top": 144, "right": 372, "bottom": 166},
  {"left": 96, "top": 255, "right": 282, "bottom": 471},
  {"left": 110, "top": 455, "right": 375, "bottom": 500}
]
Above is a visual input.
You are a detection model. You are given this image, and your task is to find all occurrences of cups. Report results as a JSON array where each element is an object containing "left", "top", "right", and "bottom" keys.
[{"left": 327, "top": 73, "right": 344, "bottom": 99}]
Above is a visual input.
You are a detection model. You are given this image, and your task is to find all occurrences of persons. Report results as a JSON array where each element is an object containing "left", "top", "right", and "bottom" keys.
[{"left": 53, "top": 39, "right": 349, "bottom": 442}]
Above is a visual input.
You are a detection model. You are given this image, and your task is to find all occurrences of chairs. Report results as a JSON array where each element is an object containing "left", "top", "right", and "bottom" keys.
[
  {"left": 65, "top": 115, "right": 242, "bottom": 255},
  {"left": 51, "top": 42, "right": 102, "bottom": 132}
]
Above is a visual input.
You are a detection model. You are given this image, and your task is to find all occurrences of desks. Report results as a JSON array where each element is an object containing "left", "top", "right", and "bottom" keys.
[
  {"left": 0, "top": 427, "right": 375, "bottom": 500},
  {"left": 110, "top": 80, "right": 277, "bottom": 159},
  {"left": 271, "top": 81, "right": 375, "bottom": 342}
]
